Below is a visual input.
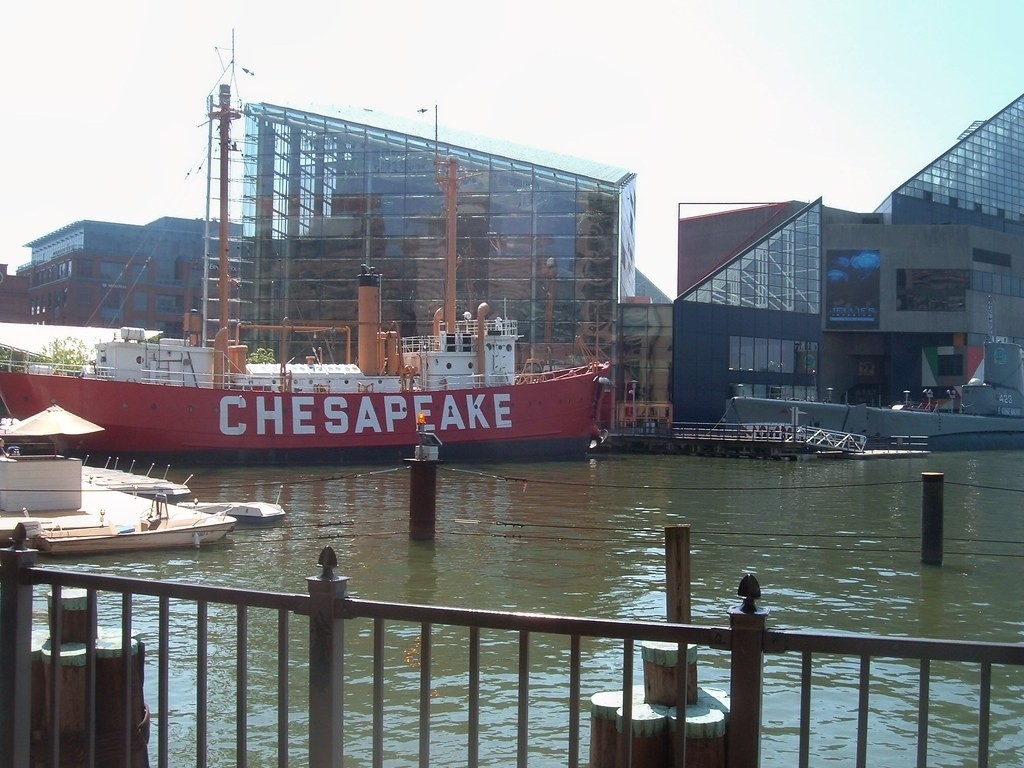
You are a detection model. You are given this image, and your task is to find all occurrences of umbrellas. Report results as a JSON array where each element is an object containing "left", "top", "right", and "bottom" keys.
[{"left": 8, "top": 404, "right": 105, "bottom": 460}]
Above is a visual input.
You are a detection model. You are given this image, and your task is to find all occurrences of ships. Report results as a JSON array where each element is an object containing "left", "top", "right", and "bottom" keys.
[{"left": 0, "top": 28, "right": 615, "bottom": 466}]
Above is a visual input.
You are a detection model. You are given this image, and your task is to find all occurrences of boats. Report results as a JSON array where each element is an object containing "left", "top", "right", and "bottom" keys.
[
  {"left": 82, "top": 455, "right": 194, "bottom": 496},
  {"left": 35, "top": 513, "right": 238, "bottom": 554},
  {"left": 176, "top": 501, "right": 285, "bottom": 523}
]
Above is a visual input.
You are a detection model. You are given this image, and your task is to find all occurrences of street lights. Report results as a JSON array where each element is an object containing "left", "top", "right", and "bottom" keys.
[
  {"left": 903, "top": 389, "right": 911, "bottom": 407},
  {"left": 629, "top": 379, "right": 640, "bottom": 436},
  {"left": 826, "top": 388, "right": 834, "bottom": 401}
]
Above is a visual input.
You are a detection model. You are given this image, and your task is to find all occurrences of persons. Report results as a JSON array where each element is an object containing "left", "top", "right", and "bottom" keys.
[
  {"left": 79, "top": 360, "right": 99, "bottom": 378},
  {"left": 0, "top": 438, "right": 7, "bottom": 456}
]
